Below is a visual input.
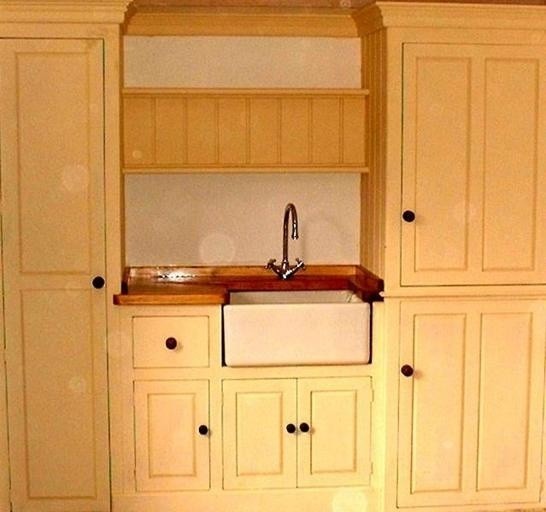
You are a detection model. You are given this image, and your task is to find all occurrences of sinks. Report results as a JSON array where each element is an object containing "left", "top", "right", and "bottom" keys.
[{"left": 223, "top": 287, "right": 371, "bottom": 368}]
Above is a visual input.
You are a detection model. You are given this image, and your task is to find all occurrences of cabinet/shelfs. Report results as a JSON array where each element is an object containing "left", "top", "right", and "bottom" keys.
[
  {"left": 217, "top": 365, "right": 375, "bottom": 494},
  {"left": 0, "top": 3, "right": 126, "bottom": 511},
  {"left": 116, "top": 304, "right": 223, "bottom": 511},
  {"left": 361, "top": 3, "right": 544, "bottom": 511}
]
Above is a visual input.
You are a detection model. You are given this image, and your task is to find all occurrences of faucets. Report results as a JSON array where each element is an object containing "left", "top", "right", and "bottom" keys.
[{"left": 263, "top": 203, "right": 306, "bottom": 278}]
